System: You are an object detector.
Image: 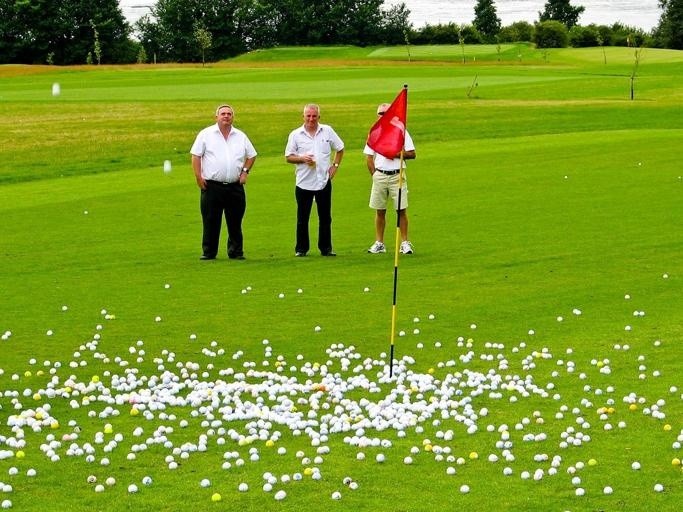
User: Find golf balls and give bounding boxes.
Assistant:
[1,271,682,509]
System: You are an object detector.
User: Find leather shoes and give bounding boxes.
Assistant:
[320,251,336,256]
[294,252,305,256]
[199,256,215,260]
[229,256,245,260]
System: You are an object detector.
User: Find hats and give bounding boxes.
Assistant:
[376,103,390,114]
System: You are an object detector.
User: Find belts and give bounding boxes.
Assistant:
[206,180,239,186]
[375,168,399,175]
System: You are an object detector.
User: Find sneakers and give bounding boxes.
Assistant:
[399,241,413,254]
[367,243,385,254]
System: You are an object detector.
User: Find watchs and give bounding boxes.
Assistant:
[333,162,339,168]
[242,167,249,174]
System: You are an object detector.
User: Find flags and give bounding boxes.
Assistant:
[367,87,405,160]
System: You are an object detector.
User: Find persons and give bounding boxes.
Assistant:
[363,102,416,253]
[284,103,345,257]
[190,105,257,260]
